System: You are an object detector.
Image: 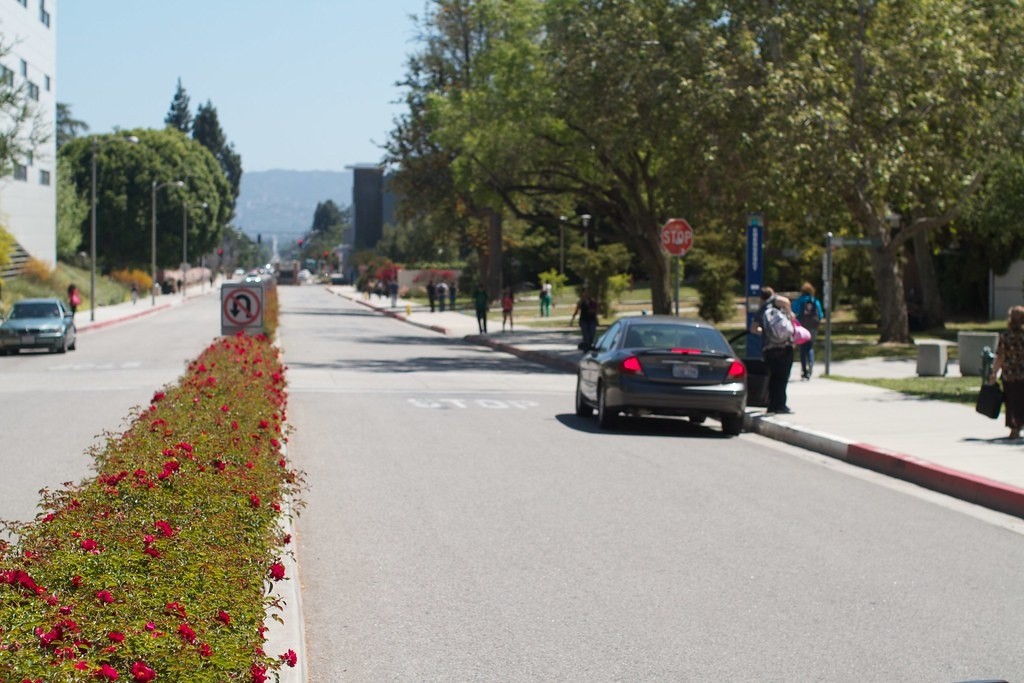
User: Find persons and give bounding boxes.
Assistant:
[751,287,795,414]
[68,284,78,316]
[502,286,514,332]
[989,306,1024,439]
[539,279,552,318]
[473,283,490,334]
[427,280,456,307]
[574,288,598,353]
[791,283,823,380]
[131,283,138,304]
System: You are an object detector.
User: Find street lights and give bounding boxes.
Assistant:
[559,216,568,274]
[152,180,184,306]
[90,136,139,322]
[582,214,591,249]
[182,203,208,294]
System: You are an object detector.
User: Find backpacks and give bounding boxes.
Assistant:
[758,297,794,343]
[796,296,820,328]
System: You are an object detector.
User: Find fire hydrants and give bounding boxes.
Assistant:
[980,346,997,383]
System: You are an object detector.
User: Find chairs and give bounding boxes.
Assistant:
[625,332,646,347]
[680,335,700,348]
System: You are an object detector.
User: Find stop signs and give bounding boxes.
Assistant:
[660,218,693,256]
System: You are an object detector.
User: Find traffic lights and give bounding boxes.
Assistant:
[218,249,223,256]
[299,238,302,248]
[324,252,329,257]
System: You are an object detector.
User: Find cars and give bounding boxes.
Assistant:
[0,298,76,358]
[574,317,769,436]
[234,269,276,282]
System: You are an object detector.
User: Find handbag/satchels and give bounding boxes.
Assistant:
[976,372,1004,419]
[790,317,811,345]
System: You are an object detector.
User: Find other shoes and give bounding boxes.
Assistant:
[767,406,795,414]
